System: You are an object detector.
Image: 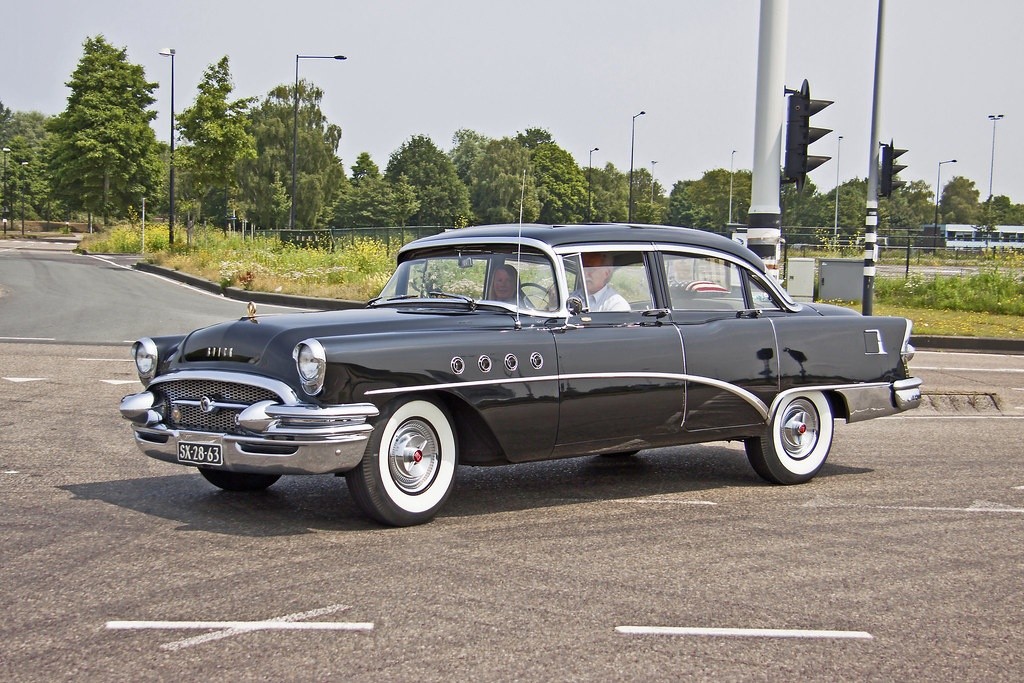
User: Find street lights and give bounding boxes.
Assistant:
[651,160,657,205]
[289,55,349,230]
[933,159,958,257]
[628,111,645,223]
[728,149,737,224]
[835,136,843,244]
[588,148,599,222]
[3,148,11,234]
[986,114,1005,256]
[22,162,28,235]
[158,48,175,245]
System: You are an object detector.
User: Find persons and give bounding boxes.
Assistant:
[545,252,631,313]
[489,264,527,307]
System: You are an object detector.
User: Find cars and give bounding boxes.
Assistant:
[117,224,923,528]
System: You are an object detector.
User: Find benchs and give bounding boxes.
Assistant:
[650,299,745,308]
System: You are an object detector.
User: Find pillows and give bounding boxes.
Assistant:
[669,279,730,295]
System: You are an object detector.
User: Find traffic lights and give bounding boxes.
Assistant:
[880,138,908,199]
[787,79,835,194]
